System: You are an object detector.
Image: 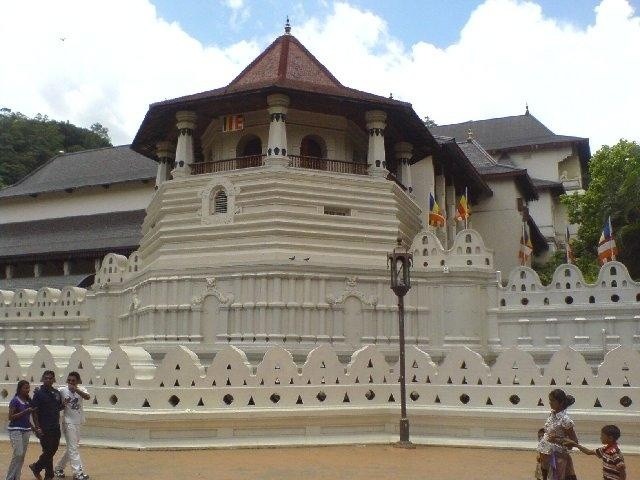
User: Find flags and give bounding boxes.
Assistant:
[429,192,445,229]
[565,229,576,259]
[520,227,533,265]
[597,219,616,261]
[457,193,472,221]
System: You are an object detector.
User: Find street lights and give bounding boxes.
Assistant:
[386,236,415,450]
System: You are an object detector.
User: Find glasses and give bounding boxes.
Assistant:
[67,378,75,382]
[44,377,53,380]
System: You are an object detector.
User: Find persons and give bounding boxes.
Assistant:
[6,380,38,480]
[30,371,90,480]
[563,424,627,480]
[536,389,579,480]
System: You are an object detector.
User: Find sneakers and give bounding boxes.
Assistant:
[72,474,88,480]
[29,463,65,480]
